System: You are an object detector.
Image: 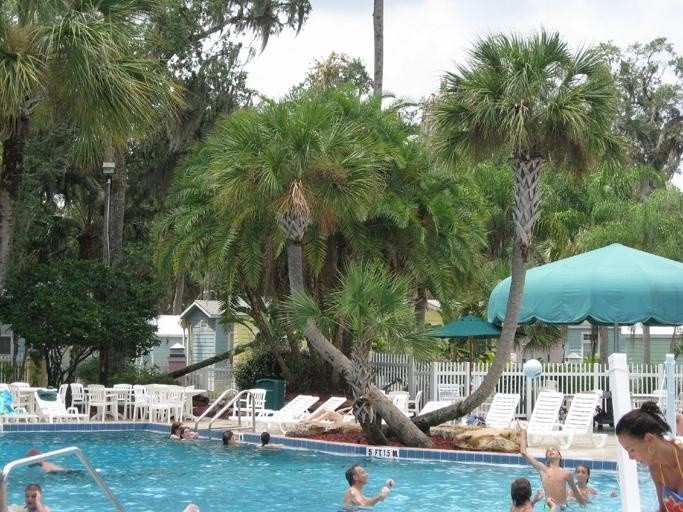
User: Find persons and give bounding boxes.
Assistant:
[169,421,183,440]
[507,477,545,512]
[179,427,199,441]
[614,402,683,511]
[569,463,617,502]
[25,449,85,476]
[222,431,238,444]
[515,416,588,510]
[7,483,51,511]
[259,432,283,448]
[309,406,360,424]
[342,464,393,507]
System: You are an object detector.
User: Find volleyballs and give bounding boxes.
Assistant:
[523,359,542,378]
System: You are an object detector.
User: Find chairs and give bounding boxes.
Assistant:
[0,378,207,430]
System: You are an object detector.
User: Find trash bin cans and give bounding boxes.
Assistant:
[256,378,284,410]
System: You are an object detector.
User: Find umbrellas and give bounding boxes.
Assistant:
[486,238,681,352]
[416,310,526,398]
